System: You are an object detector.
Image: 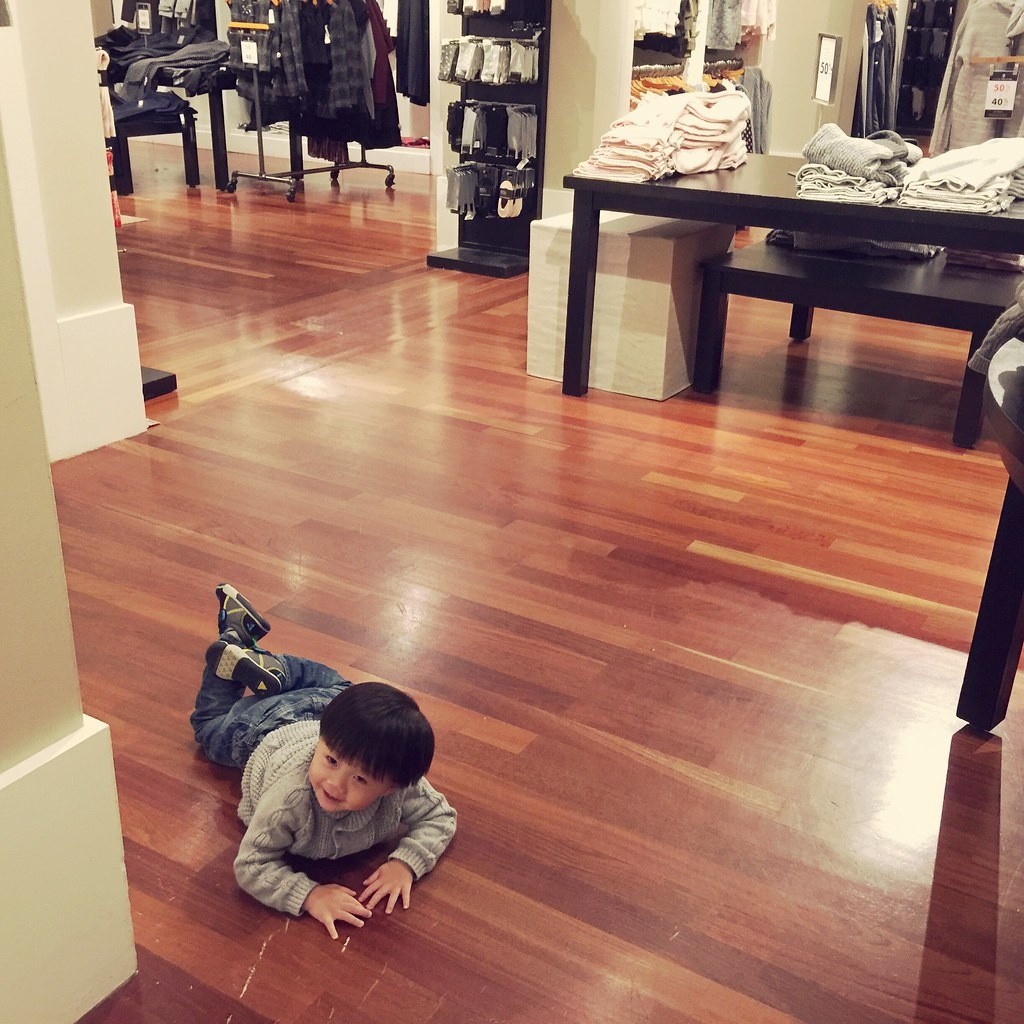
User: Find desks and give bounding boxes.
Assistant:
[561,151,1024,398]
[108,59,305,192]
[954,328,1024,734]
[105,92,202,195]
[693,234,1024,450]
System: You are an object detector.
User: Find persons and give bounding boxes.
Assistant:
[189,581,456,940]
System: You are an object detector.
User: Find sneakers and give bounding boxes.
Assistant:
[215,583,271,650]
[205,638,288,698]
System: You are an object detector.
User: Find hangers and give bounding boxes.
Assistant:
[630,57,760,113]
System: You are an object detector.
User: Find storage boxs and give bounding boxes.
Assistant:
[524,210,737,402]
